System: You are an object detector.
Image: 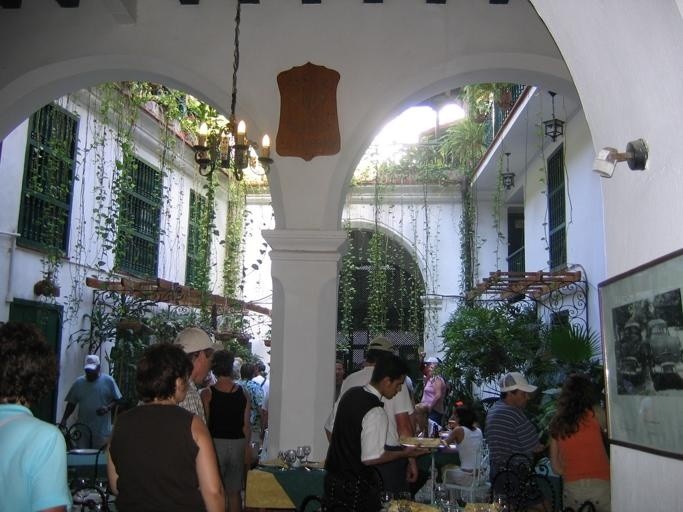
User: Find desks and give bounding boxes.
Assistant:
[397,437,447,482]
[256,464,327,511]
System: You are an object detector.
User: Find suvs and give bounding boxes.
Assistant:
[646,318,680,364]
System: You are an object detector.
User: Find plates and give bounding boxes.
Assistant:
[404,443,445,448]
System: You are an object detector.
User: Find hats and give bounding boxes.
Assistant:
[82,354,102,371]
[364,334,396,356]
[496,369,539,397]
[173,327,225,355]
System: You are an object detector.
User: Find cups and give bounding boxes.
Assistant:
[494,495,507,512]
[434,483,449,511]
[303,445,311,465]
[287,450,297,468]
[379,491,394,511]
[397,491,411,512]
[296,446,305,469]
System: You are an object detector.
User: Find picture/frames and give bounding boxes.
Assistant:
[597,249,683,462]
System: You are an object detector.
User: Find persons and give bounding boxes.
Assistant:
[548,376,610,512]
[234,364,265,491]
[394,348,416,408]
[415,403,443,438]
[171,328,213,426]
[201,350,255,512]
[106,342,228,512]
[341,337,418,500]
[0,320,73,512]
[419,352,446,427]
[232,359,241,384]
[484,372,551,512]
[59,355,123,450]
[254,363,269,437]
[234,357,244,369]
[335,358,346,403]
[324,355,432,512]
[448,401,464,430]
[442,406,484,512]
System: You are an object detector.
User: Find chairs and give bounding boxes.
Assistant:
[56,422,69,450]
[437,445,490,510]
[498,450,565,511]
[69,420,91,450]
[94,440,110,483]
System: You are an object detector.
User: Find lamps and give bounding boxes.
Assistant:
[499,148,516,192]
[543,90,568,141]
[592,137,651,178]
[191,1,273,182]
